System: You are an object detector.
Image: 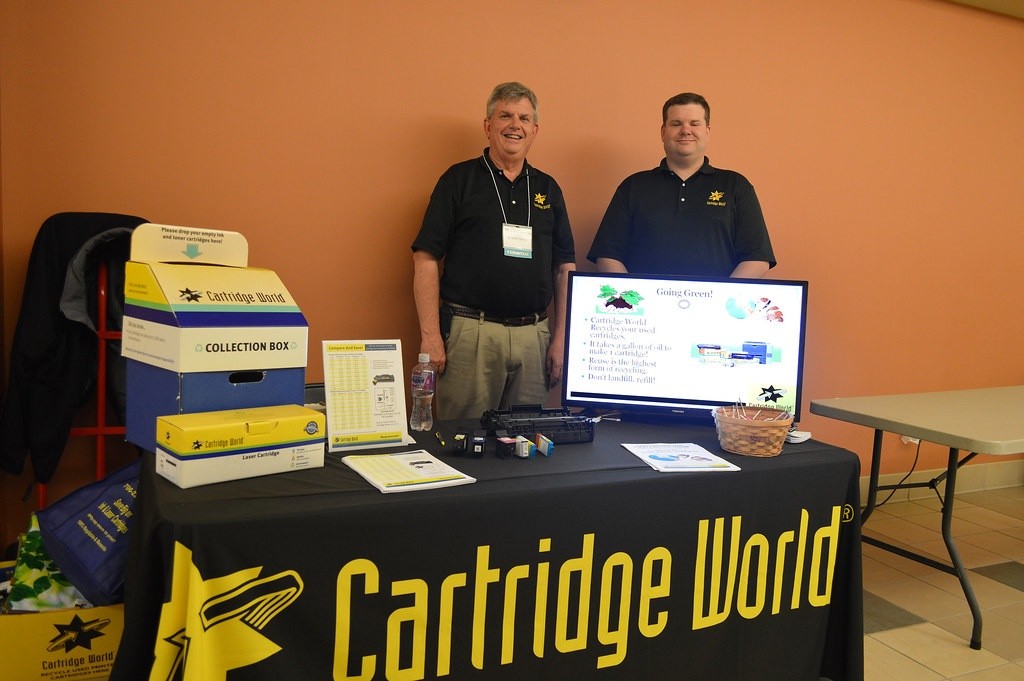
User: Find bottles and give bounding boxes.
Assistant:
[409,353,436,431]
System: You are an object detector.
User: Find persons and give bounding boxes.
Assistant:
[411,82,577,421]
[585,93,777,279]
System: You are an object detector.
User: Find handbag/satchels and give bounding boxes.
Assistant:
[35,461,139,606]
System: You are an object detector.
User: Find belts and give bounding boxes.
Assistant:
[448,305,548,327]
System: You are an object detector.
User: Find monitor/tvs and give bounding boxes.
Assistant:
[561,270,809,426]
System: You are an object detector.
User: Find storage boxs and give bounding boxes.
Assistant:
[123,225,327,490]
[0,603,125,681]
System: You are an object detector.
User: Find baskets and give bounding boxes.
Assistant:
[712,406,795,457]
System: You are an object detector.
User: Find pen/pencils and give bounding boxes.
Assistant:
[435,432,446,446]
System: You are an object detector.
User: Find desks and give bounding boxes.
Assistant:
[106,414,865,681]
[809,385,1024,651]
